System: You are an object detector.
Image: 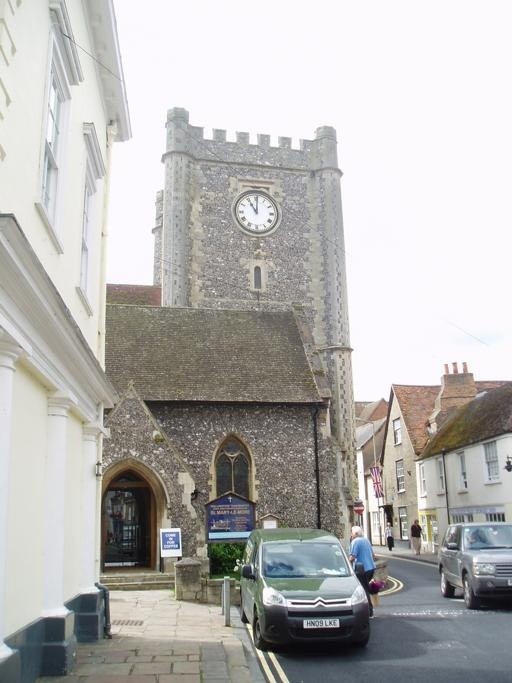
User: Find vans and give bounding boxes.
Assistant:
[235,524,372,650]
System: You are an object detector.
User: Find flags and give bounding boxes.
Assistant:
[369,466,385,496]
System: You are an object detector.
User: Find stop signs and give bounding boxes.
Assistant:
[353,502,365,514]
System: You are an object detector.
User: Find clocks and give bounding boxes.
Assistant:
[230,189,282,238]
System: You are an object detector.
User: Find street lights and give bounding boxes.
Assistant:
[355,416,384,547]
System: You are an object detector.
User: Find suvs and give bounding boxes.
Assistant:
[435,521,512,607]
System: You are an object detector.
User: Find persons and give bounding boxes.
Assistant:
[384,521,394,551]
[409,519,422,555]
[464,531,486,547]
[347,525,376,622]
